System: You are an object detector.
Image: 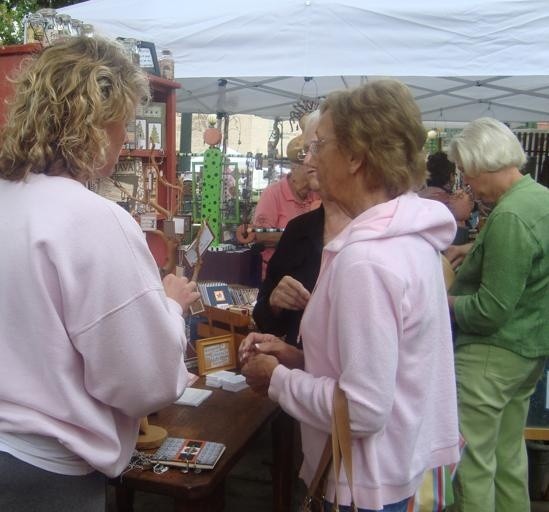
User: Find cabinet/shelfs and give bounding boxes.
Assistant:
[194,303,252,351]
[0,43,184,279]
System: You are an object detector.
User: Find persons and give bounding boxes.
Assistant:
[0,33,200,512]
[233,78,549,512]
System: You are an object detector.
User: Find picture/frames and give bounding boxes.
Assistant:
[195,332,237,375]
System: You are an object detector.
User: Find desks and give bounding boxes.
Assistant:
[105,372,291,512]
[179,242,264,289]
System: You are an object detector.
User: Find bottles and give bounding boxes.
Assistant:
[160,50,175,81]
[122,37,141,65]
[24,7,95,46]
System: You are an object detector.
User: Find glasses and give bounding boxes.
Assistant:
[297,143,320,162]
